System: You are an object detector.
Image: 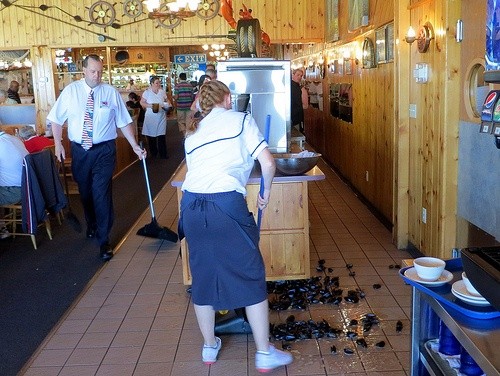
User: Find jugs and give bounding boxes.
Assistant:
[151,103,159,113]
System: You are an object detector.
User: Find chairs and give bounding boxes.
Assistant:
[0,125,73,251]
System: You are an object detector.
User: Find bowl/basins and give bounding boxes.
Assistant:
[413,256,446,281]
[462,271,482,296]
[438,318,484,376]
[162,106,175,111]
[271,152,322,175]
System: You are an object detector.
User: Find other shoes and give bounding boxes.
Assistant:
[86,226,94,237]
[100,241,113,260]
[0,221,10,239]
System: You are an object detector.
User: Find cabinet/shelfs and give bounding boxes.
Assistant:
[400,258,500,376]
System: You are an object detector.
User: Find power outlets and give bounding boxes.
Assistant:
[422,207,427,224]
[365,170,368,182]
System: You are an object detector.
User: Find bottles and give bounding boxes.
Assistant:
[14,128,19,138]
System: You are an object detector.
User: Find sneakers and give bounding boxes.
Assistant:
[201,335,221,364]
[256,345,292,372]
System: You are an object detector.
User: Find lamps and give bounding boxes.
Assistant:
[142,0,201,19]
[404,26,430,53]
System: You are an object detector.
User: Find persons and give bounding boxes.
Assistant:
[47,54,146,260]
[126,93,145,128]
[179,80,293,374]
[0,121,30,238]
[126,79,140,92]
[291,70,308,129]
[139,77,172,160]
[190,64,216,117]
[0,90,18,104]
[18,125,53,153]
[174,73,193,135]
[7,81,21,104]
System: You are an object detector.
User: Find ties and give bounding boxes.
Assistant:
[81,89,94,151]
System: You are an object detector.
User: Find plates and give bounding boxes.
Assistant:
[450,280,490,307]
[404,267,454,287]
